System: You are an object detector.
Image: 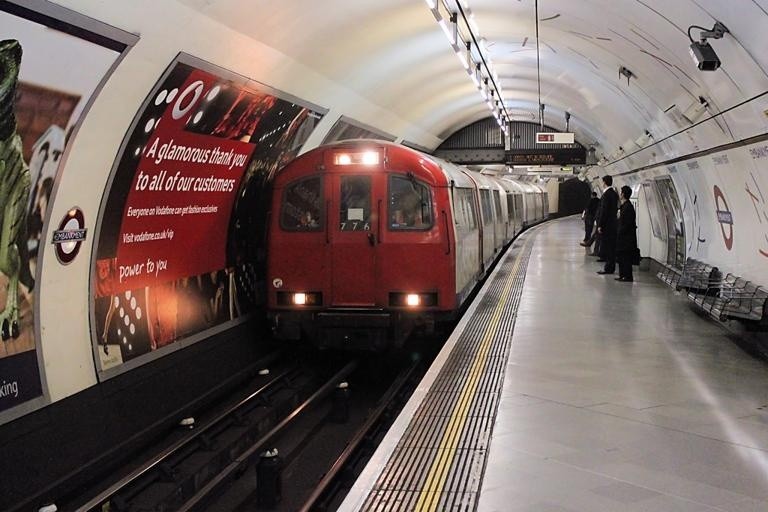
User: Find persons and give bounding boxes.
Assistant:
[580,191,600,241]
[580,228,600,256]
[596,175,619,275]
[390,189,428,230]
[614,184,637,282]
[200,269,227,325]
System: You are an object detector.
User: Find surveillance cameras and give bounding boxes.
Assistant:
[688,40,722,72]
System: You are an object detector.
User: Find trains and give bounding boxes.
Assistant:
[266,138,549,351]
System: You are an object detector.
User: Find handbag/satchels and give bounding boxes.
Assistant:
[615,247,640,265]
[580,237,594,246]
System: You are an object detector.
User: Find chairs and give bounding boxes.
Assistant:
[656,256,767,333]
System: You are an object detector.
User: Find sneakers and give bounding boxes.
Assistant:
[589,251,633,281]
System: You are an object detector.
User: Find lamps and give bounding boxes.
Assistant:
[580,133,652,175]
[682,96,709,124]
[425,0,510,137]
